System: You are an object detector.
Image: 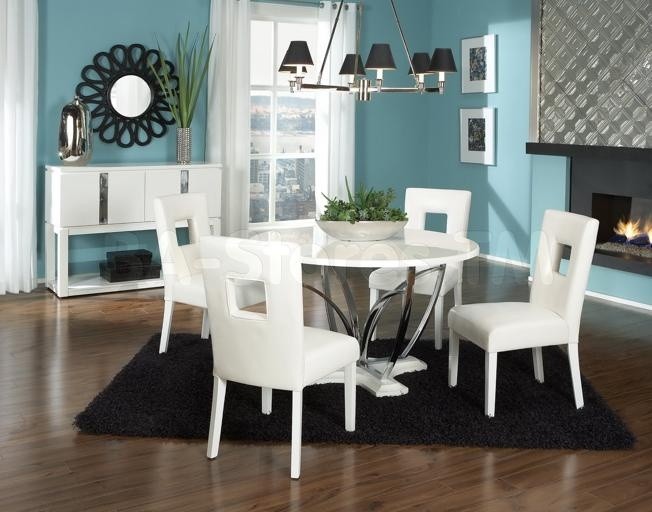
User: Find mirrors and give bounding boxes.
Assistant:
[73,43,183,148]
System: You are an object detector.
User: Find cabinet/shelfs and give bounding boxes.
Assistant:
[43,158,225,301]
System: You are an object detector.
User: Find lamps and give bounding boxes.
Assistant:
[274,0,460,105]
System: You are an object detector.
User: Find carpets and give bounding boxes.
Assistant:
[66,324,639,460]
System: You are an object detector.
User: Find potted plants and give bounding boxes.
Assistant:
[145,16,225,167]
[308,175,411,244]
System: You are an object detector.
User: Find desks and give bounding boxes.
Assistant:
[244,222,482,403]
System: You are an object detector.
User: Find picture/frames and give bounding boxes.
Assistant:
[456,107,500,169]
[454,32,497,96]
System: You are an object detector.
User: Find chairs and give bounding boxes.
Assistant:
[191,233,364,480]
[359,183,476,350]
[439,205,603,420]
[144,188,226,357]
